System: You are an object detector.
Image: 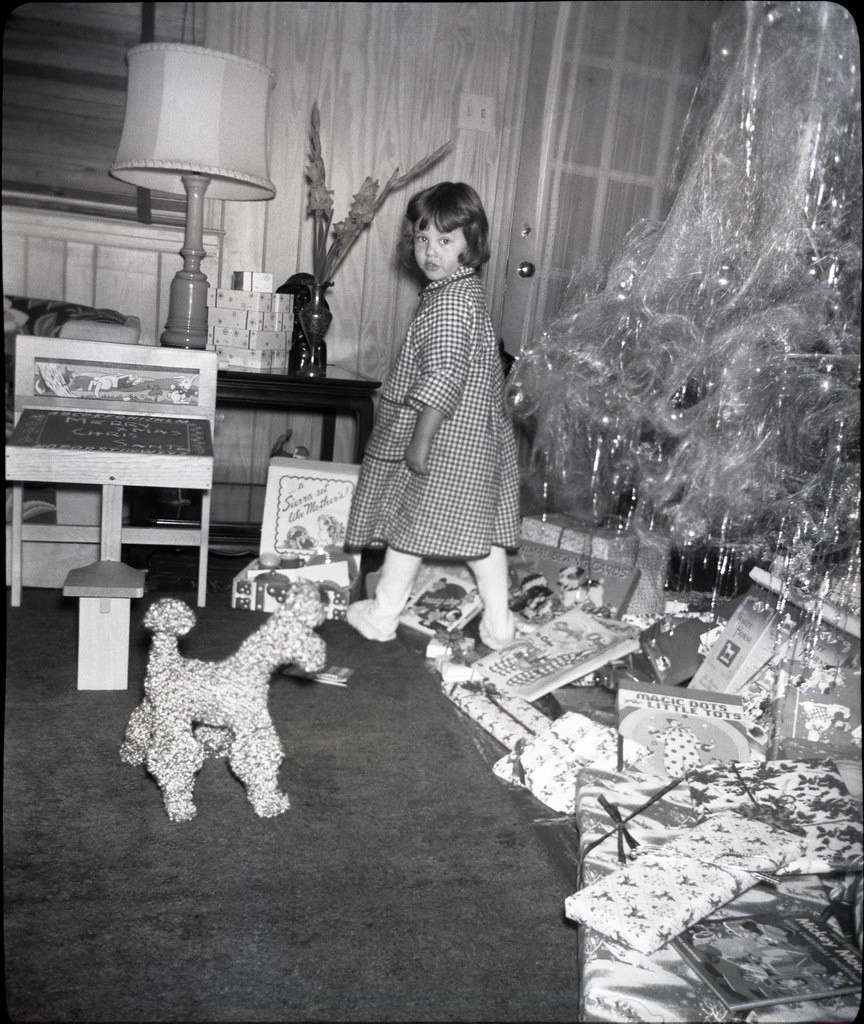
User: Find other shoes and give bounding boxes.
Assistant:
[344,598,397,642]
[478,608,516,650]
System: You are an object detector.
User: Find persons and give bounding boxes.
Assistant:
[343,180,525,652]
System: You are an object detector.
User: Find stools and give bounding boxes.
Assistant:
[5,409,213,608]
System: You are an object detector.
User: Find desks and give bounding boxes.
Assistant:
[124,360,386,587]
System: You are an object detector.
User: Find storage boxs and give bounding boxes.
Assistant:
[421,601,864,1024]
[229,458,361,622]
[206,272,294,373]
[524,515,674,618]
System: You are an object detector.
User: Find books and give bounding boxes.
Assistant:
[687,591,858,720]
[470,604,642,704]
[613,676,752,773]
[398,573,485,641]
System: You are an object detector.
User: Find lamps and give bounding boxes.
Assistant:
[109,43,278,349]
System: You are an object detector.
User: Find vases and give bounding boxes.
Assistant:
[298,282,335,377]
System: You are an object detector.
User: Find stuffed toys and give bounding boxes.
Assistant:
[554,564,606,612]
[118,583,328,822]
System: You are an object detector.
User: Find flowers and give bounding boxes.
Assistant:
[300,102,454,283]
